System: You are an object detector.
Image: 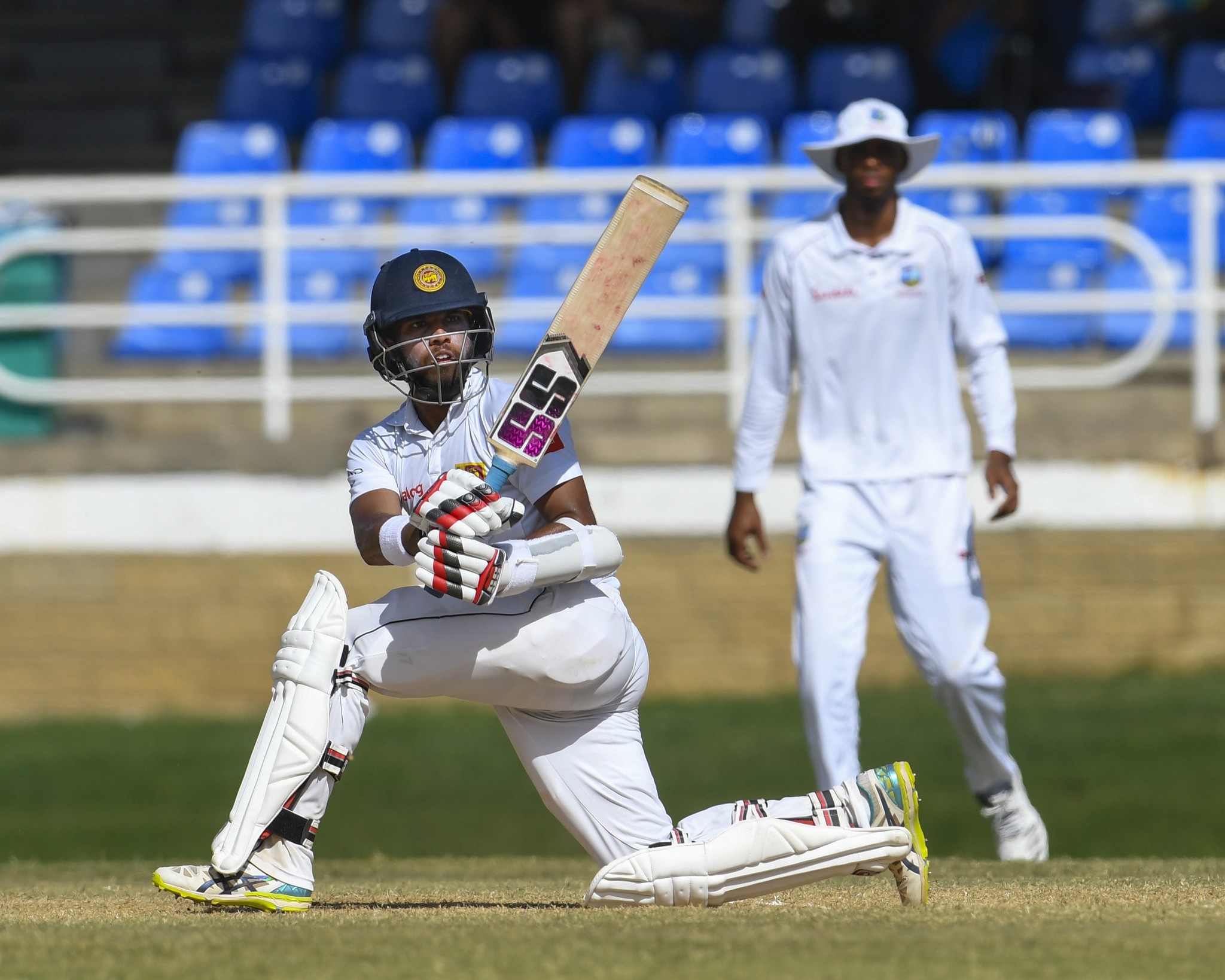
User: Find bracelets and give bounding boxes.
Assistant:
[379,516,416,567]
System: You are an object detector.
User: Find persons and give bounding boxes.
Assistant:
[727,99,1049,861]
[152,248,929,912]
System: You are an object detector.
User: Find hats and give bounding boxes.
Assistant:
[804,98,941,185]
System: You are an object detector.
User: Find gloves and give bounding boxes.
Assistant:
[414,529,506,608]
[408,469,513,538]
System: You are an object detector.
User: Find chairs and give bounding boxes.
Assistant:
[100,2,1224,365]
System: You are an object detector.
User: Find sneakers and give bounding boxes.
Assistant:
[858,761,932,913]
[977,752,1048,865]
[150,858,312,916]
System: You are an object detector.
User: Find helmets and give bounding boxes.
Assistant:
[362,248,492,380]
